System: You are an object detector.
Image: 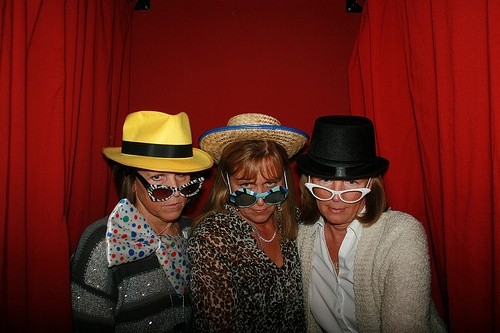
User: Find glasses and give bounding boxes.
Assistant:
[305,174,372,203]
[227,171,289,206]
[129,170,205,201]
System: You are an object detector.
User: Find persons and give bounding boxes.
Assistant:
[68,110,215,333]
[185,112,311,333]
[294,114,451,333]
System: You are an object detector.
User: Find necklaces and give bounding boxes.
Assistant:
[250,225,277,243]
[151,222,172,236]
[323,224,340,271]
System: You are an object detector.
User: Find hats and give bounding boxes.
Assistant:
[302,116,389,179]
[103,110,213,173]
[198,112,309,166]
